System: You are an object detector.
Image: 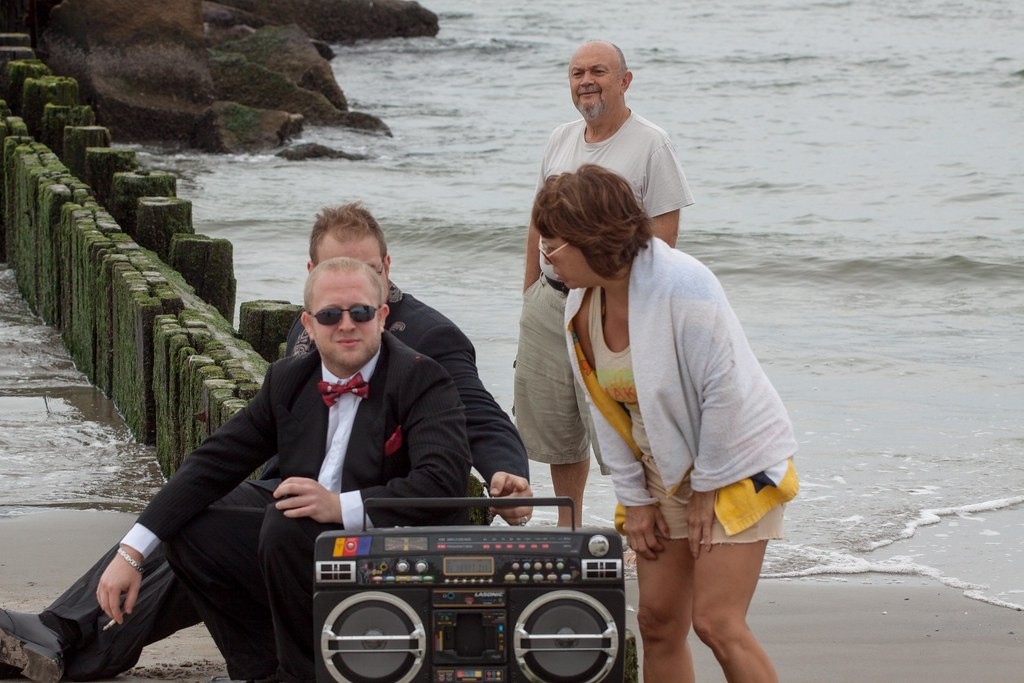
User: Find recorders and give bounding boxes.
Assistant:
[312,497,630,683]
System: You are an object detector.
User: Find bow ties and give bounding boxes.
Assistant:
[316,371,369,408]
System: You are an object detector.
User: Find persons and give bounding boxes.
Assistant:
[534,163,799,683]
[509,38,697,528]
[97,258,473,683]
[1,203,533,683]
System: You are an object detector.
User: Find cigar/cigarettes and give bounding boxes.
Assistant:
[101,610,126,630]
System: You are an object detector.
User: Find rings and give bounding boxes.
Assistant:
[520,516,527,526]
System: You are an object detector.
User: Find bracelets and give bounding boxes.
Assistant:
[116,547,146,576]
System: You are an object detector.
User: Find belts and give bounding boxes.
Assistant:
[545,274,569,296]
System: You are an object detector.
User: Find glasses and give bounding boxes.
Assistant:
[538,242,568,264]
[313,303,378,325]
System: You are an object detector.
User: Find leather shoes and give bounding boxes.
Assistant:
[0,603,74,683]
